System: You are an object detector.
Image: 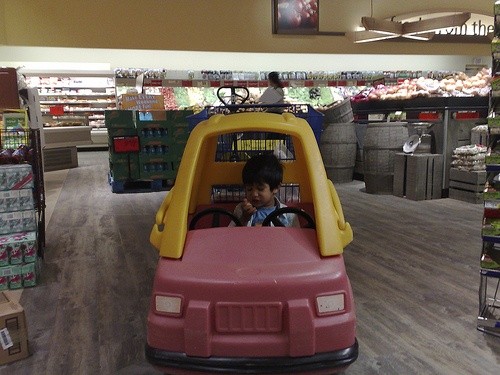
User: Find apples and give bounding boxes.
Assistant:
[368,67,491,101]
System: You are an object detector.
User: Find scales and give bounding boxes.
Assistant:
[402,121,432,153]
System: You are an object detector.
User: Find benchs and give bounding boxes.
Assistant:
[193,202,317,229]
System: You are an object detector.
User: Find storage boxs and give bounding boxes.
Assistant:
[0,289,30,365]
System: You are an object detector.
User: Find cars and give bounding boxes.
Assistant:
[139,110,360,367]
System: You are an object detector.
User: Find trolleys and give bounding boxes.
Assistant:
[217,84,250,112]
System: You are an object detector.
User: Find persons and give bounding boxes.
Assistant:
[261,72,284,114]
[227,154,300,230]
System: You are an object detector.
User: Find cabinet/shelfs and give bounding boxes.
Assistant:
[0,0,500,337]
